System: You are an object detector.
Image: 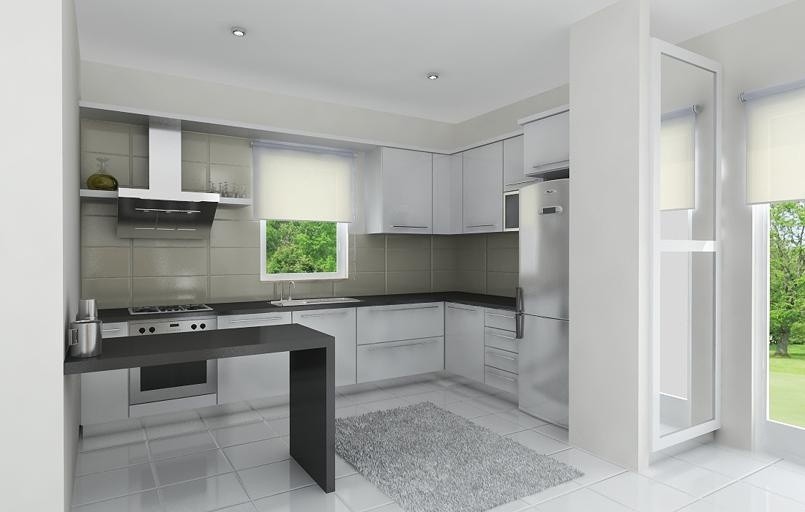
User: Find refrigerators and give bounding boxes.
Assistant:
[514,177,569,432]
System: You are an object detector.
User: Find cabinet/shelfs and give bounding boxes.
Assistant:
[517,104,571,179]
[485,327,520,356]
[357,335,444,384]
[357,145,434,235]
[484,346,519,376]
[484,365,519,395]
[445,302,485,383]
[80,321,128,426]
[217,312,292,405]
[432,152,464,234]
[485,306,517,333]
[502,134,545,196]
[292,307,356,387]
[356,301,445,346]
[462,140,504,234]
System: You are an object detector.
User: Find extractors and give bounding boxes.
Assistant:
[116,187,220,241]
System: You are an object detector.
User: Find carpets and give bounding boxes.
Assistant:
[334,400,585,512]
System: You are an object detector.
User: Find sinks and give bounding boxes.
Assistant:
[268,298,361,306]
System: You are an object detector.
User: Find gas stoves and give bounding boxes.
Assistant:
[132,306,157,313]
[182,304,205,310]
[158,305,180,312]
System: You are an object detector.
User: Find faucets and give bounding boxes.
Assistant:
[287,280,295,302]
